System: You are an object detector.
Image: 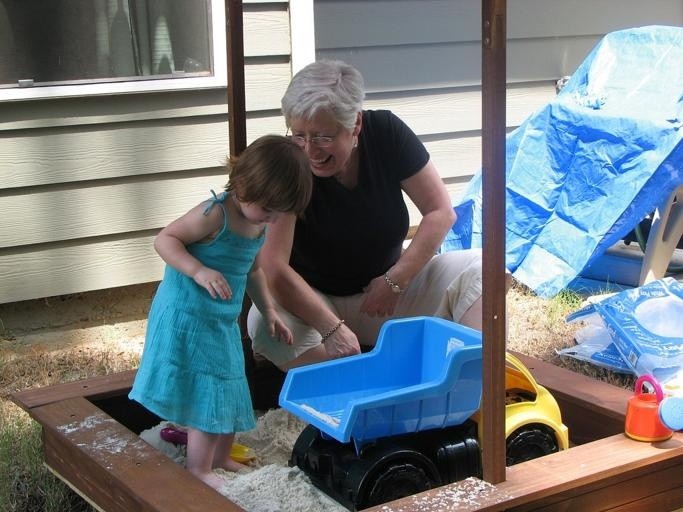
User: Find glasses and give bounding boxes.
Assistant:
[286,125,342,148]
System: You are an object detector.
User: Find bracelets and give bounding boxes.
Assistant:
[321,315,347,341]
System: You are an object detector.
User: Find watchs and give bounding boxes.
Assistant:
[384,274,404,297]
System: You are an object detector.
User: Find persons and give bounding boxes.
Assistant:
[128,134,318,476]
[242,57,512,392]
